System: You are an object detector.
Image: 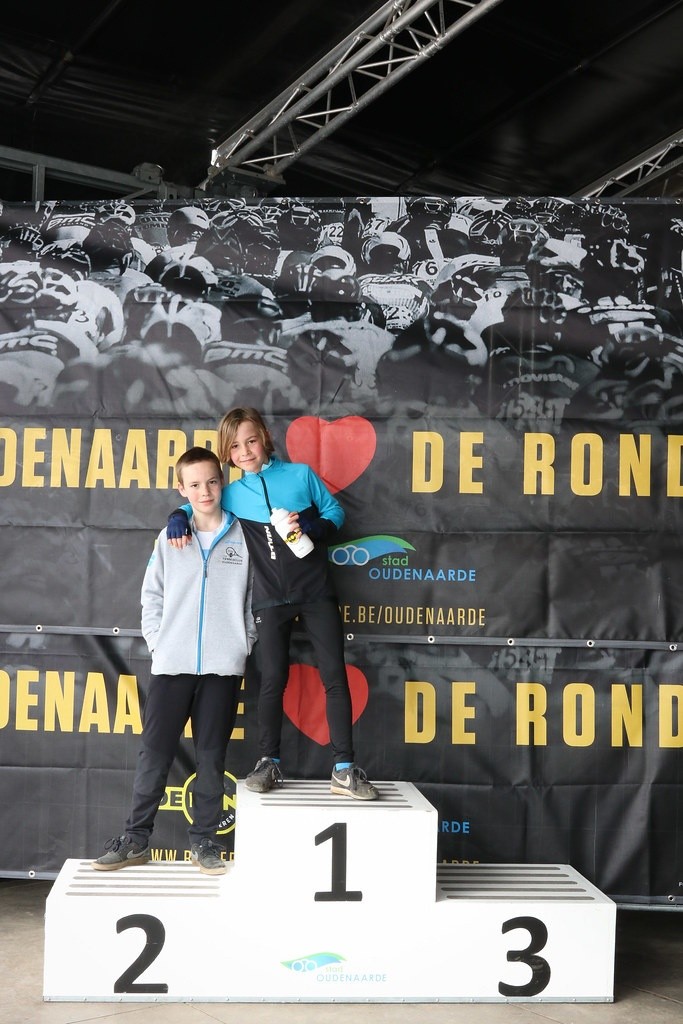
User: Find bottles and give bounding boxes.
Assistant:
[268,506,315,558]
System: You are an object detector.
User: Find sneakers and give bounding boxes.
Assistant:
[330,766,379,800]
[245,760,278,792]
[93,836,149,871]
[190,842,226,875]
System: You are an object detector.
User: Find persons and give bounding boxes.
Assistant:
[1,194,682,439]
[164,405,379,802]
[94,447,258,872]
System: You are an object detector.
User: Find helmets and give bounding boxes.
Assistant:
[0,201,682,428]
